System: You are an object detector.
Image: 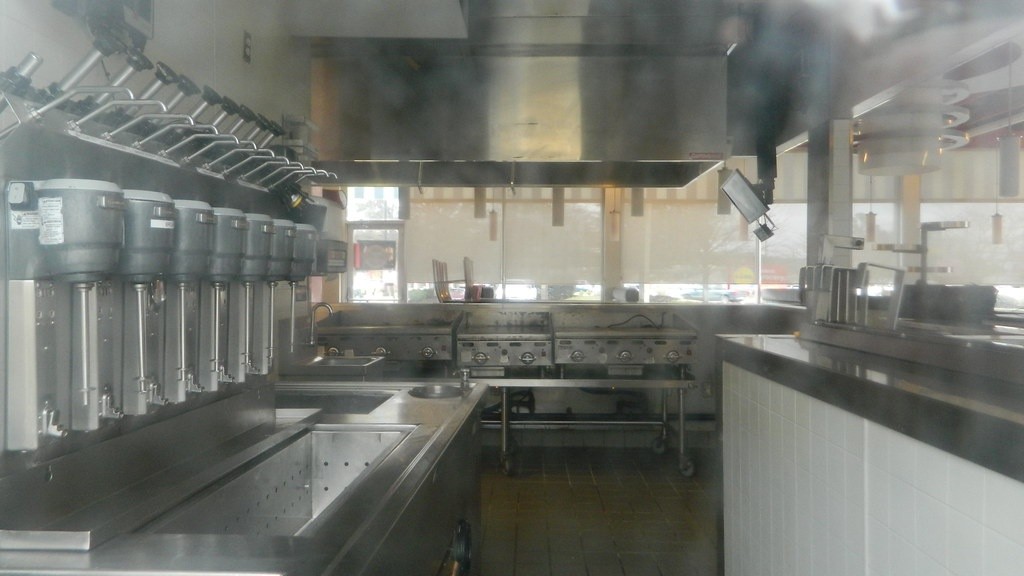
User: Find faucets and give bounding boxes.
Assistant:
[310,301,334,348]
[452,367,471,391]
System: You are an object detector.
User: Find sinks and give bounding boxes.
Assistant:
[407,382,463,401]
[132,423,420,539]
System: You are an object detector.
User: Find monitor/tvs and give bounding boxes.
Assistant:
[723,168,767,226]
[356,239,396,271]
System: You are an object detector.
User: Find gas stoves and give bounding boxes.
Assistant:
[549,301,698,368]
[453,309,552,368]
[315,304,462,362]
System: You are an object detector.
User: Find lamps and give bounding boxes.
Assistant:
[861,110,943,174]
